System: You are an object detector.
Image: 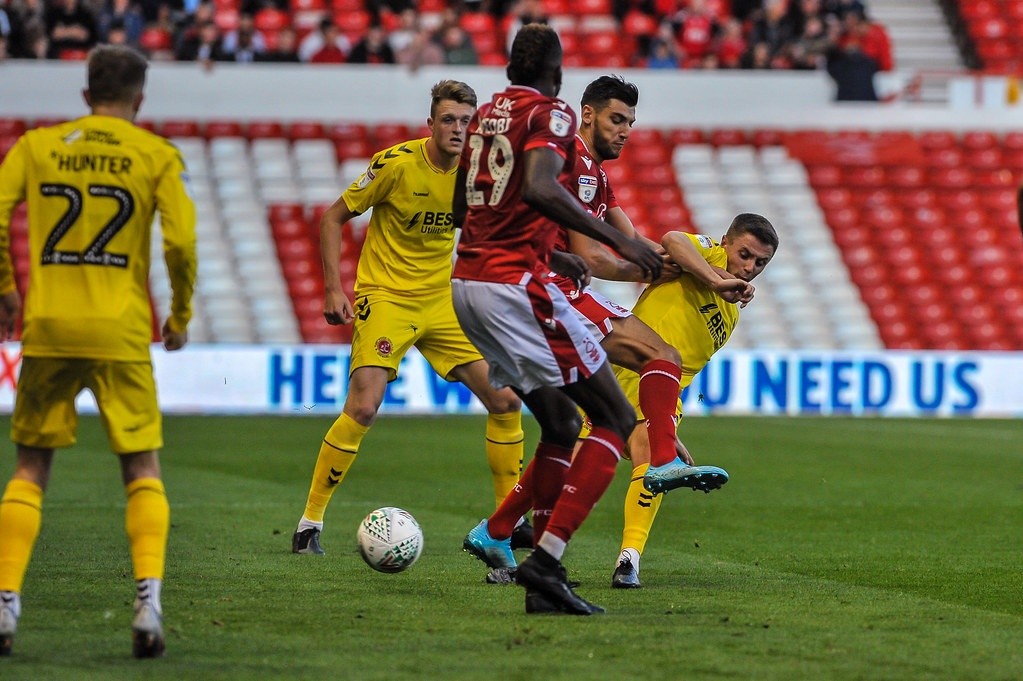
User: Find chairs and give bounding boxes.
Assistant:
[60,0,1023,74]
[0,114,1023,352]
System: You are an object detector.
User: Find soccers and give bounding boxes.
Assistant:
[357,507,424,574]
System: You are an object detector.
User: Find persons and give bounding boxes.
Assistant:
[465,73,730,583]
[0,47,196,660]
[289,79,535,557]
[452,23,662,618]
[0,0,899,105]
[573,212,780,589]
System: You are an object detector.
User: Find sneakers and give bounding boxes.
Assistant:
[463,519,517,569]
[131,603,166,659]
[612,558,641,588]
[643,456,729,497]
[293,527,326,556]
[487,569,516,584]
[516,552,593,615]
[526,589,605,615]
[0,595,20,654]
[511,519,539,549]
